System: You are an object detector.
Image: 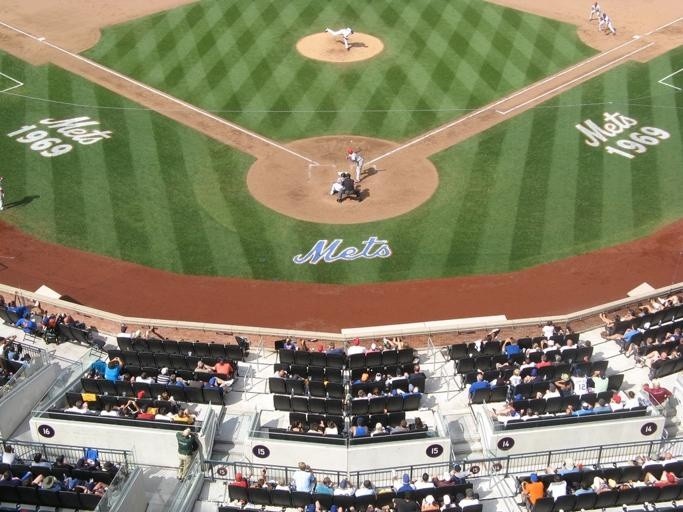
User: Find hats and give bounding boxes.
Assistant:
[644,322,650,329]
[161,367,169,375]
[531,474,538,482]
[340,480,346,488]
[653,381,659,386]
[615,396,621,402]
[209,378,215,385]
[403,465,461,483]
[562,373,570,381]
[56,455,116,472]
[138,391,145,398]
[183,428,191,436]
[306,504,337,512]
[236,473,242,480]
[425,489,474,505]
[44,476,54,489]
[666,473,675,483]
[316,339,377,352]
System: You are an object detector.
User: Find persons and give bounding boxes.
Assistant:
[469,321,640,425]
[272,339,428,438]
[330,172,346,195]
[336,173,362,202]
[598,13,616,35]
[0,294,236,498]
[517,454,680,509]
[599,294,682,367]
[325,27,353,48]
[589,2,600,20]
[347,149,364,181]
[232,461,479,511]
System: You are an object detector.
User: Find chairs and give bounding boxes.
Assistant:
[0,286,97,387]
[1,461,116,509]
[47,336,247,435]
[446,331,648,432]
[267,346,429,449]
[514,458,682,510]
[608,303,682,381]
[218,479,486,510]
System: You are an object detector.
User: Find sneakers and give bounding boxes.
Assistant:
[518,503,526,506]
[225,379,234,393]
